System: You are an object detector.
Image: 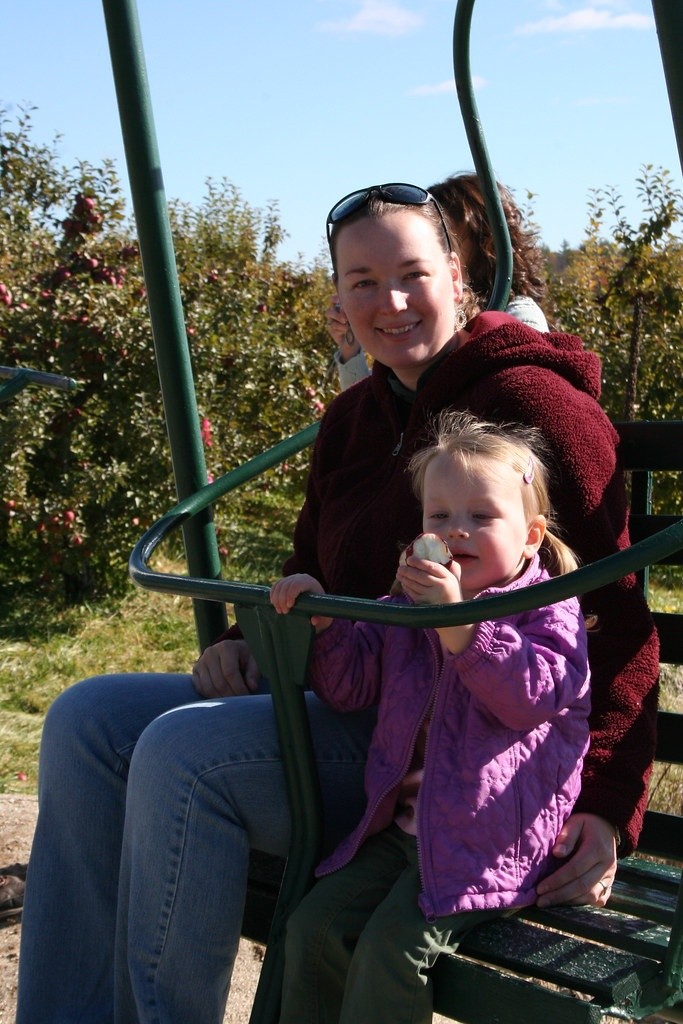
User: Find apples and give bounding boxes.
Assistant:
[397,532,453,571]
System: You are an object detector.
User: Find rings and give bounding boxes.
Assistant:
[600,880,608,895]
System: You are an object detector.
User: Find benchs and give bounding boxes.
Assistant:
[237,426,683,1024]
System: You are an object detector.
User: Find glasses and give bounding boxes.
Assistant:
[325,183,452,256]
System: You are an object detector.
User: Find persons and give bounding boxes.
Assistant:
[326,172,550,393]
[270,411,590,1024]
[13,183,661,1024]
